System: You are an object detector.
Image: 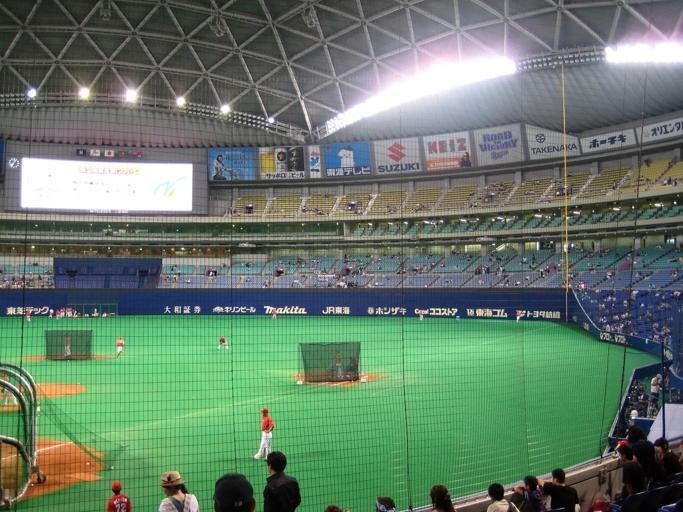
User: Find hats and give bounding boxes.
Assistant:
[258,407,268,414]
[110,480,123,492]
[158,470,186,490]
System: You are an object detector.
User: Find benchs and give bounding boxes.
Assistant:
[351,205,683,237]
[1,219,344,240]
[230,156,683,219]
[0,263,51,289]
[160,246,683,289]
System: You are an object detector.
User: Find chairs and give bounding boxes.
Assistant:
[575,291,683,348]
[604,471,683,511]
[0,153,683,290]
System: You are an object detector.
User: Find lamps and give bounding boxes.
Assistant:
[204,9,226,40]
[98,2,114,22]
[300,4,317,31]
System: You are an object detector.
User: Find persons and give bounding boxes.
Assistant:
[211,155,232,180]
